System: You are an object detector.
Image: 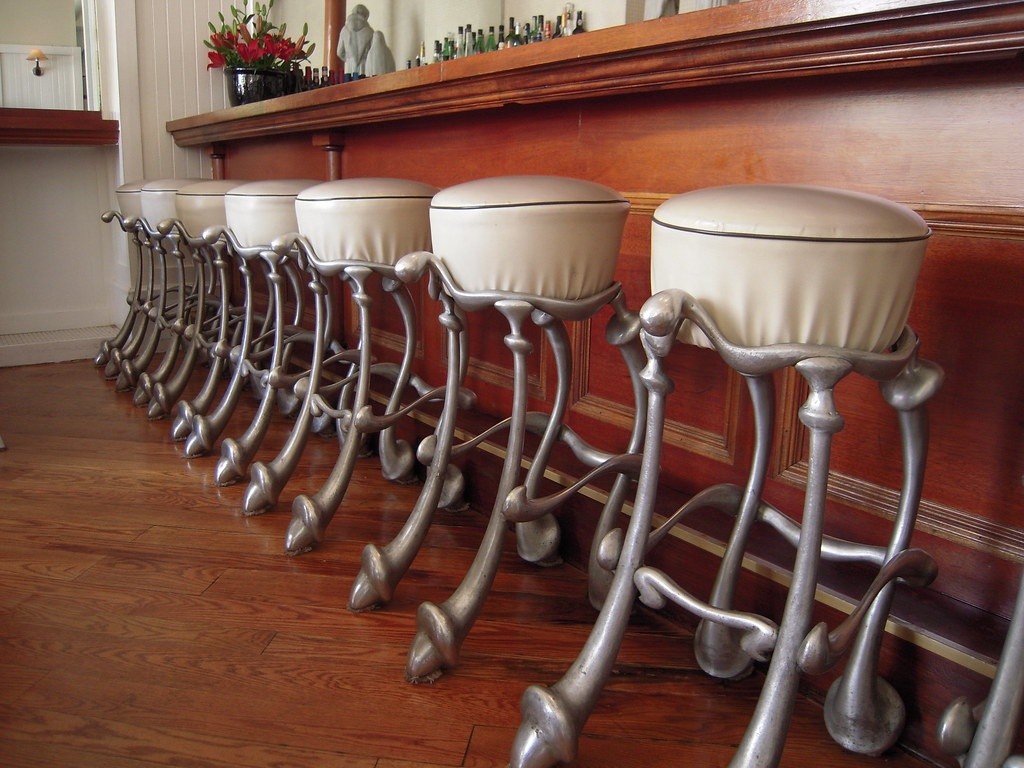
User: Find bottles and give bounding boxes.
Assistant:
[292,4,586,91]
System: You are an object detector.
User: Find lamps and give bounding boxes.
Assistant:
[24,48,48,76]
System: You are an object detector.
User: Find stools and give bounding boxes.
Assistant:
[96,175,946,768]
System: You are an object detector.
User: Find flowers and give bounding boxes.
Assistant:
[203,0,316,76]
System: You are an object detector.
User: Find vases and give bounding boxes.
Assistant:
[224,66,294,106]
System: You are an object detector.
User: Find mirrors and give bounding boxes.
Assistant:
[0,0,101,112]
